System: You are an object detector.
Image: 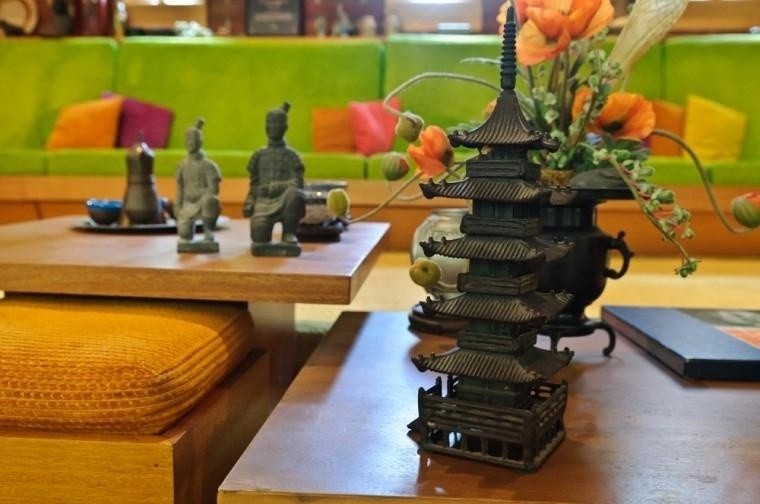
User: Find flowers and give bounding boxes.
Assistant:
[326,0,760,279]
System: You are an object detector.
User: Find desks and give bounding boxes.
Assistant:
[0,213,392,305]
[216,310,760,504]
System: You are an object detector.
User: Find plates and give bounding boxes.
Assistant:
[69,215,231,232]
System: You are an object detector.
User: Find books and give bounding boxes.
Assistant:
[601,306,760,381]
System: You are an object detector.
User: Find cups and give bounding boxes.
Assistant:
[86,198,123,226]
[303,180,347,192]
[299,190,335,223]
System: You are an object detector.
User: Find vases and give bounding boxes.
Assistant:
[534,187,641,359]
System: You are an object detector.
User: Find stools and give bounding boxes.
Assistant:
[0,294,273,504]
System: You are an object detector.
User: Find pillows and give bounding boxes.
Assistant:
[44,95,125,150]
[643,97,687,157]
[347,96,401,158]
[682,95,748,162]
[311,107,356,153]
[100,92,176,150]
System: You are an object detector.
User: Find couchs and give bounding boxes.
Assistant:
[0,33,759,258]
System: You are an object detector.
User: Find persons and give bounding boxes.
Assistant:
[175,119,221,240]
[242,100,307,244]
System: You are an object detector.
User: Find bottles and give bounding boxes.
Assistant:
[411,207,473,285]
[123,129,162,224]
